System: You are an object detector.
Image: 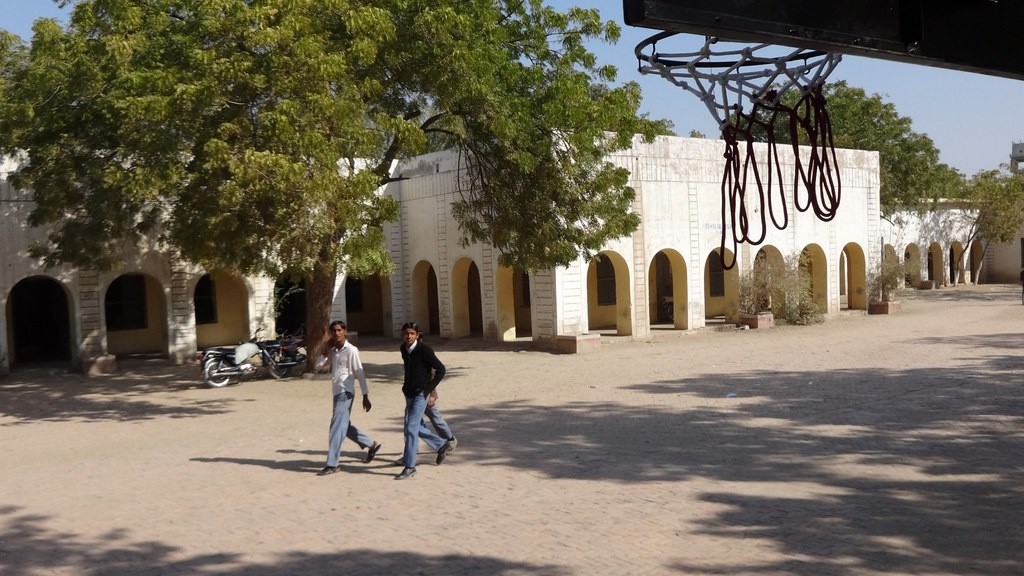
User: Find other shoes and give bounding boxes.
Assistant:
[366,441,382,463]
[448,436,457,454]
[319,466,341,476]
[394,457,405,465]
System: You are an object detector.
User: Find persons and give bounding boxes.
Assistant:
[394,322,458,480]
[316,320,381,475]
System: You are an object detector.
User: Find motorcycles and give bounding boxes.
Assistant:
[254,327,308,389]
[197,325,273,388]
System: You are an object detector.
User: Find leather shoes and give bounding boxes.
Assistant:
[397,467,417,479]
[436,441,451,464]
